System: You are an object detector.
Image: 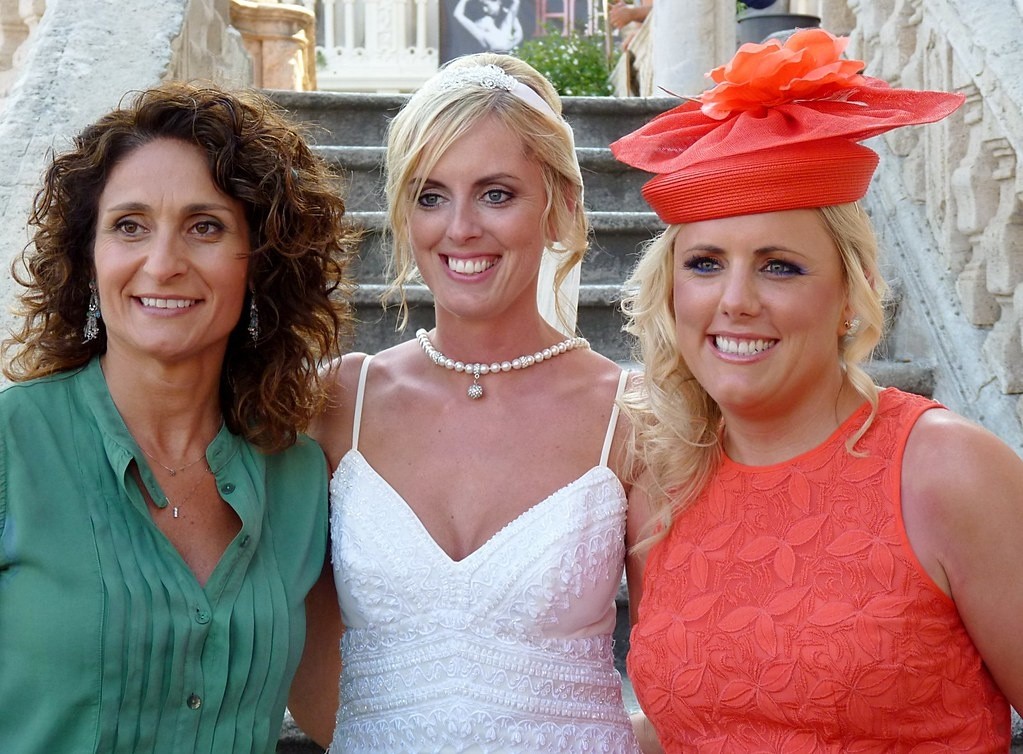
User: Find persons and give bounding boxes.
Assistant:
[623,140,1023,754]
[607,0,653,52]
[303,54,645,754]
[0,83,346,754]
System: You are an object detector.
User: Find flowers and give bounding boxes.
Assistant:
[699,27,865,120]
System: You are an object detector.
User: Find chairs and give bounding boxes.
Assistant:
[229,0,318,91]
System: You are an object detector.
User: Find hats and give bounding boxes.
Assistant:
[609,27,966,225]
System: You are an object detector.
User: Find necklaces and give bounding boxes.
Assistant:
[414,328,589,400]
[137,444,211,517]
